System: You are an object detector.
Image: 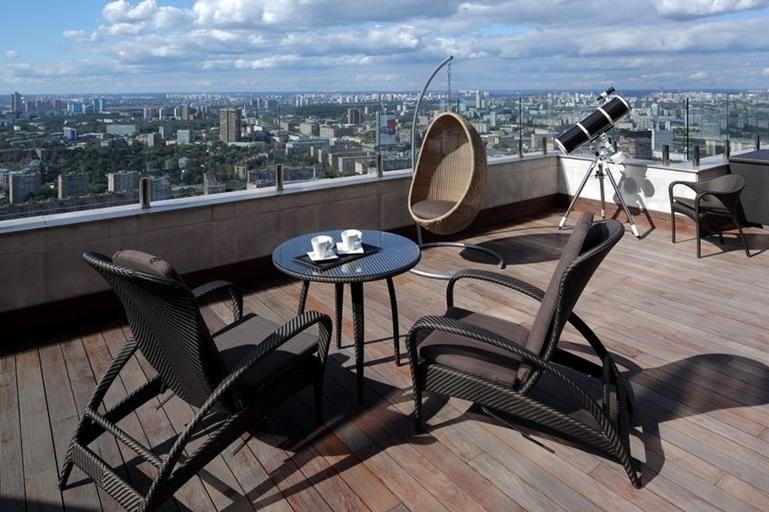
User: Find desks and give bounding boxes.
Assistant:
[272,228,420,407]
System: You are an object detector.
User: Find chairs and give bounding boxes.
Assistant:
[405,211,641,489]
[669,174,751,258]
[60,250,333,512]
[408,111,488,236]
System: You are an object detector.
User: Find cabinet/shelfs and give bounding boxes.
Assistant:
[727,150,769,225]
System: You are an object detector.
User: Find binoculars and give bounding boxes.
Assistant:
[553,87,632,155]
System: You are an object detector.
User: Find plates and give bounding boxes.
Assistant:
[336,242,364,255]
[306,251,339,262]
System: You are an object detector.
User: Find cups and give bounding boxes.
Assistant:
[310,235,333,257]
[340,230,363,250]
